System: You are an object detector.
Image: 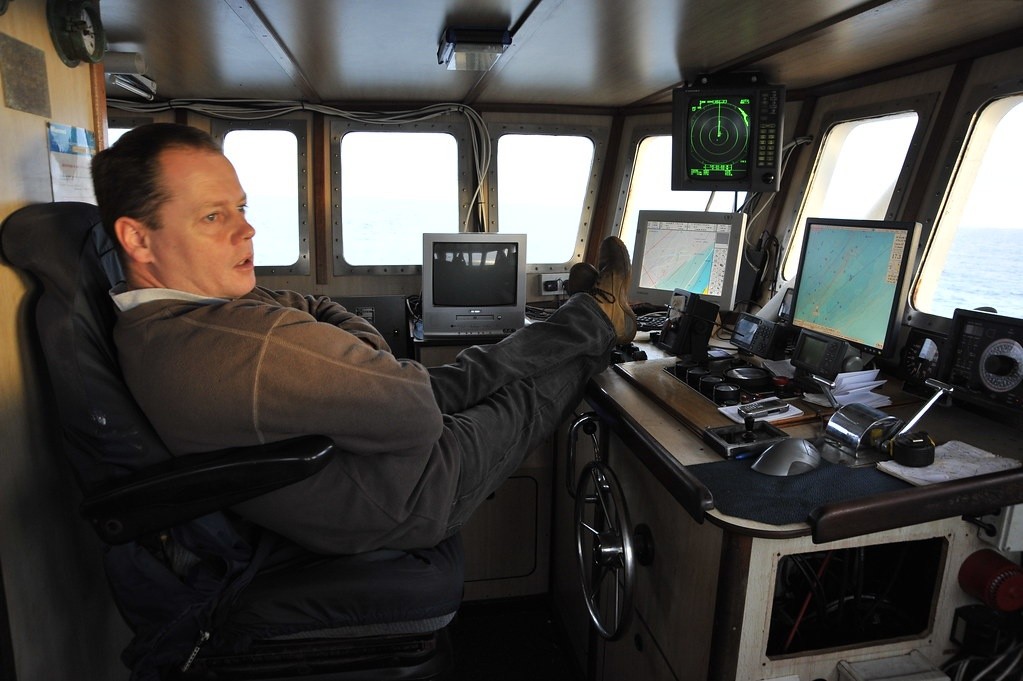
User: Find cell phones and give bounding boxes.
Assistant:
[738,399,789,419]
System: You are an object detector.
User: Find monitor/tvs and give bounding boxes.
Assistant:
[628,210,747,311]
[789,217,922,359]
[687,95,754,181]
[423,233,527,337]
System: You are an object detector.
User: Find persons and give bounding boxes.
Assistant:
[90,123,637,556]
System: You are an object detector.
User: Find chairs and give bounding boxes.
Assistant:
[0,200,467,681]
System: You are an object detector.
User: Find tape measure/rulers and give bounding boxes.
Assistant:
[889,431,936,468]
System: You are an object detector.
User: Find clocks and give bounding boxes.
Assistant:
[62,1,109,63]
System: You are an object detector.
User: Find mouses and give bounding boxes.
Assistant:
[750,437,822,476]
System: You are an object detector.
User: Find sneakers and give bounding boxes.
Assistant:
[567,263,599,298]
[583,236,637,345]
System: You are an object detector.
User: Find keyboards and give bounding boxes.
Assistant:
[636,317,667,330]
